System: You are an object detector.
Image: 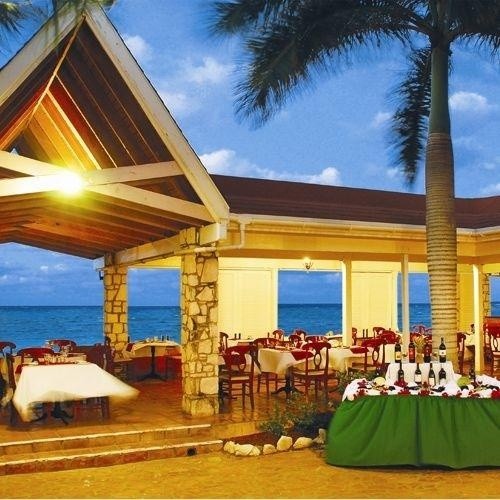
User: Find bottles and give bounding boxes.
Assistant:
[397,362,405,381]
[438,335,447,363]
[395,337,402,362]
[408,337,415,362]
[428,362,438,386]
[439,363,446,386]
[423,338,431,363]
[414,362,422,384]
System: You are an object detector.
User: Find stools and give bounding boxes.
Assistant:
[165,355,182,379]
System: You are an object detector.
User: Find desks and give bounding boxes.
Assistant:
[125,341,178,383]
[326,375,500,467]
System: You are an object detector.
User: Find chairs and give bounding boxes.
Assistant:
[482,321,500,375]
[219,325,398,412]
[1,338,132,430]
[411,324,432,355]
[458,331,466,372]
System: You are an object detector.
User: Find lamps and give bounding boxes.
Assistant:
[304,261,313,269]
